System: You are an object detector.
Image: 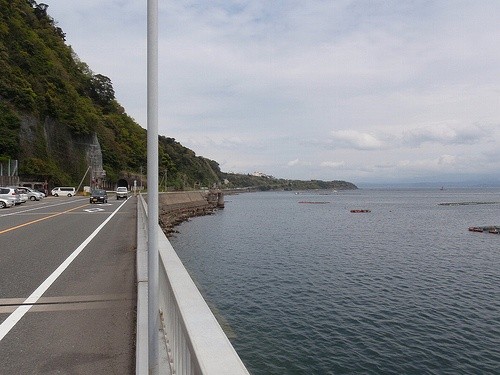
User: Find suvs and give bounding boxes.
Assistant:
[0,187,21,209]
[116,187,127,199]
[17,187,46,201]
[90,189,107,203]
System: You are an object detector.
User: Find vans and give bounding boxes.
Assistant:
[51,187,76,197]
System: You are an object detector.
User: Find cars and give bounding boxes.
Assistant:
[17,189,29,202]
